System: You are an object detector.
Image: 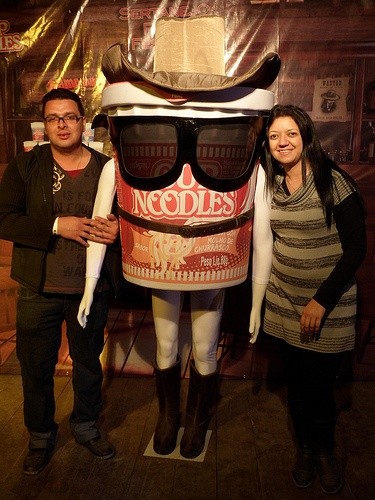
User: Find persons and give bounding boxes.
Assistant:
[0,88,119,475]
[259,104,368,494]
[77,43,281,459]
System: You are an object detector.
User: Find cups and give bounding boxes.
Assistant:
[82,122,104,153]
[101,82,275,290]
[23,121,51,152]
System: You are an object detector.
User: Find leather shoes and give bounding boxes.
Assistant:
[83,437,114,459]
[22,448,54,475]
[292,446,317,487]
[315,454,342,493]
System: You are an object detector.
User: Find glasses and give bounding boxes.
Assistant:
[43,113,83,123]
